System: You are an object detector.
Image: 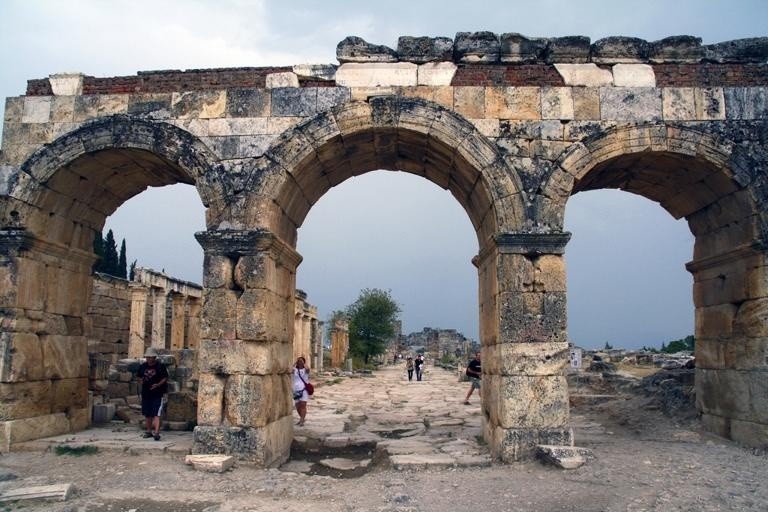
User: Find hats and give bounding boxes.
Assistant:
[143,347,158,358]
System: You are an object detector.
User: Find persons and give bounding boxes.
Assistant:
[133,346,171,443]
[415,355,424,382]
[290,357,313,427]
[391,351,426,364]
[463,351,482,406]
[405,357,414,382]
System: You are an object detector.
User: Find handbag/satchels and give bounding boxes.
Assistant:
[306,383,314,395]
[292,390,303,399]
